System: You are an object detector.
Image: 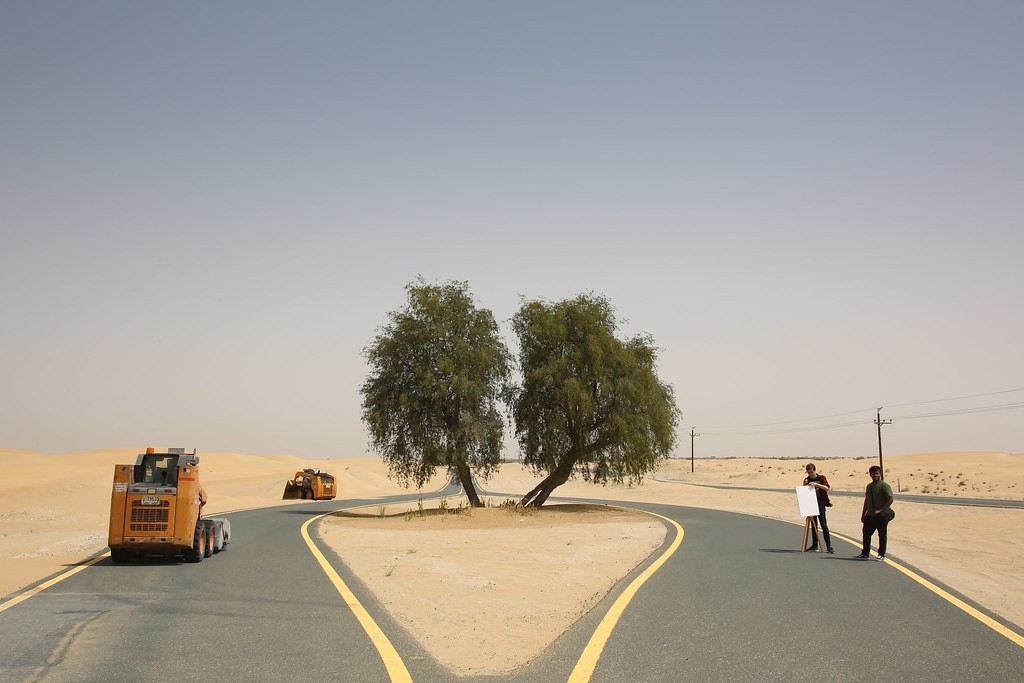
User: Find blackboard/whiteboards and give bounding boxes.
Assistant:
[795,485,820,517]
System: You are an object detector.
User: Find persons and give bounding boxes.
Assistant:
[302,473,316,500]
[803,463,835,554]
[853,466,895,561]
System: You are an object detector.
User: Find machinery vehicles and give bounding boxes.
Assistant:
[108,445,232,563]
[289,467,338,502]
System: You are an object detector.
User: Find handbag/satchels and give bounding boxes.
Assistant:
[876,507,896,524]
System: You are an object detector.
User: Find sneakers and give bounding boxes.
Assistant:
[805,545,820,552]
[874,554,885,561]
[827,545,836,554]
[853,554,868,560]
